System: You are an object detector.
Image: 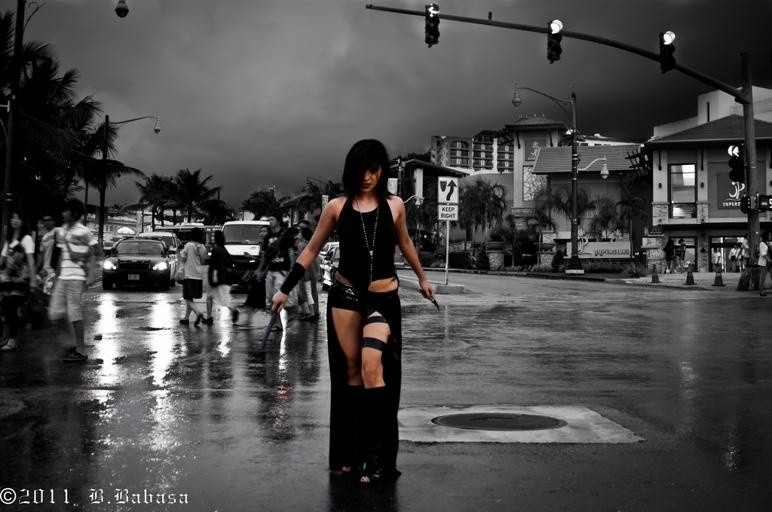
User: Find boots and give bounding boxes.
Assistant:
[341,383,388,483]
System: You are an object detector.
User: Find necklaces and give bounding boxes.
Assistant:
[356,197,379,288]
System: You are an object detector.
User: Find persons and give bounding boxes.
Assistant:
[663,240,679,273]
[202,229,239,325]
[1,205,38,353]
[174,227,208,325]
[43,197,94,362]
[242,225,272,326]
[296,227,319,323]
[38,211,62,279]
[712,232,771,296]
[677,238,687,272]
[272,138,443,487]
[258,210,296,332]
[295,220,322,317]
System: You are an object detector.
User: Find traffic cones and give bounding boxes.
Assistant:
[682,262,698,286]
[711,265,727,287]
[648,263,663,285]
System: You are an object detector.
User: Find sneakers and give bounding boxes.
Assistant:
[232,309,239,321]
[180,313,212,326]
[62,349,89,362]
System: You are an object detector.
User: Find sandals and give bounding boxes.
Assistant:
[3,341,16,350]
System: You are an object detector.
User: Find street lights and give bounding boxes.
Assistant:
[1,1,130,243]
[510,79,609,276]
[403,195,426,203]
[306,175,331,207]
[91,111,164,260]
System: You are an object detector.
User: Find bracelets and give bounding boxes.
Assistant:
[279,262,306,295]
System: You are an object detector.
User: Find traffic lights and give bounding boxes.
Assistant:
[424,3,440,48]
[755,193,772,213]
[658,30,676,75]
[545,18,565,65]
[727,141,747,182]
[740,194,755,213]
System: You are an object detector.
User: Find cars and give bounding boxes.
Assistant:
[104,219,340,290]
